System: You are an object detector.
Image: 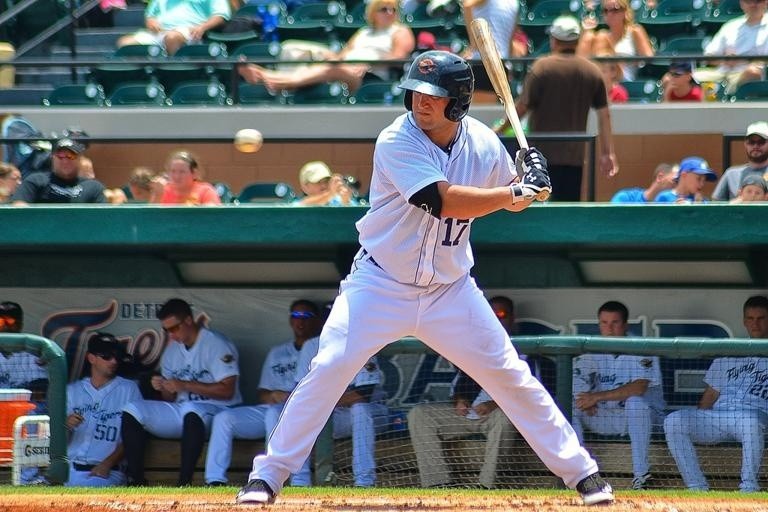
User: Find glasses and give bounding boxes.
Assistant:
[164,322,183,333]
[496,311,506,319]
[95,353,120,361]
[54,153,77,160]
[376,7,397,12]
[601,8,623,13]
[291,311,313,320]
[746,139,766,146]
[0,318,15,326]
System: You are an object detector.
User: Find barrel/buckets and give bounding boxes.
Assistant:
[0,388,35,469]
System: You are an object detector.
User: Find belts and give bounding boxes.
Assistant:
[363,249,382,270]
[72,462,120,472]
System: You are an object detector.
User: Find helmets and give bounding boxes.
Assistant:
[397,51,475,122]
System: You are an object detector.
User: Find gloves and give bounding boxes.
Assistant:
[516,147,548,182]
[511,169,553,204]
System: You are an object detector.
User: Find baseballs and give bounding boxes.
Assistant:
[234,128,261,153]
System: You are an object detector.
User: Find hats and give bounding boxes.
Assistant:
[298,161,332,184]
[0,301,22,318]
[52,138,82,154]
[88,335,133,360]
[666,63,692,72]
[546,17,580,42]
[678,157,717,182]
[746,123,768,139]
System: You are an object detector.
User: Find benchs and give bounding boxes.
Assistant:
[136,432,768,488]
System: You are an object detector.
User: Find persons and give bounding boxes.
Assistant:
[568,301,670,491]
[489,13,620,203]
[660,294,768,490]
[0,300,47,400]
[610,121,768,204]
[285,300,388,487]
[236,49,614,505]
[575,0,768,105]
[203,299,320,486]
[97,0,530,108]
[122,296,243,489]
[1,127,224,207]
[66,332,146,488]
[404,295,547,486]
[291,161,364,207]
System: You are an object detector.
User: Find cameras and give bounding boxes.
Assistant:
[342,176,362,190]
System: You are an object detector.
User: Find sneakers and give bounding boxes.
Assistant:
[236,478,275,504]
[577,471,614,505]
[632,476,648,490]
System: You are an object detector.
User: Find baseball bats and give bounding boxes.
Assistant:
[469,18,552,203]
